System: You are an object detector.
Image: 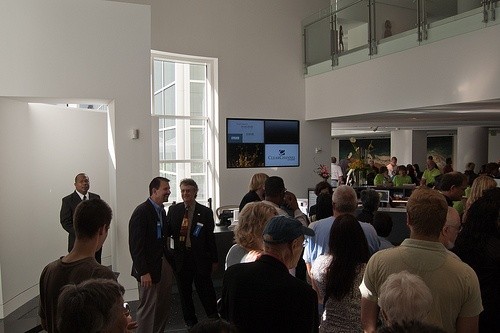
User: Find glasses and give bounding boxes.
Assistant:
[295,238,308,248]
[281,187,288,192]
[447,224,463,233]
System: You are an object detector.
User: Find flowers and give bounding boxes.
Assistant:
[317,165,331,178]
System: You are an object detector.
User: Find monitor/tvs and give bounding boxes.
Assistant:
[225,118,300,167]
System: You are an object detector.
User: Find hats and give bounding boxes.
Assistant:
[262,217,315,244]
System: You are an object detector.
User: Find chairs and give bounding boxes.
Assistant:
[216,206,240,224]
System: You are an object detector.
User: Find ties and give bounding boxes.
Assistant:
[185,208,188,236]
[83,195,87,201]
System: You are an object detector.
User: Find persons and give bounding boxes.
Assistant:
[331,155,500,188]
[221,172,500,333]
[129,177,171,333]
[358,188,483,333]
[38,199,118,333]
[310,214,373,333]
[61,173,102,264]
[378,270,449,333]
[163,179,220,333]
[190,317,237,333]
[220,215,320,333]
[55,277,138,333]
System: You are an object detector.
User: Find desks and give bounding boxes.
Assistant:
[213,225,241,252]
